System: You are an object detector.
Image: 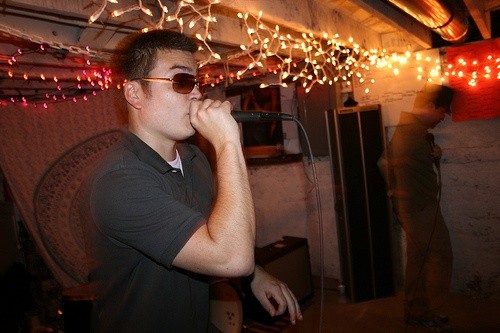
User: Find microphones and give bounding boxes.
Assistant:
[426,133,440,170]
[231,109,294,122]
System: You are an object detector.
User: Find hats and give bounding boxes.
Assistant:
[416,82,456,116]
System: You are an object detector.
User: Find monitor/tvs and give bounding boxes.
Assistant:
[239,235,315,325]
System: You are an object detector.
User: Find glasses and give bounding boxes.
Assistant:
[130,73,199,94]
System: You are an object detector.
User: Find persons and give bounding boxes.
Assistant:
[385,83,456,333]
[88,30,304,333]
